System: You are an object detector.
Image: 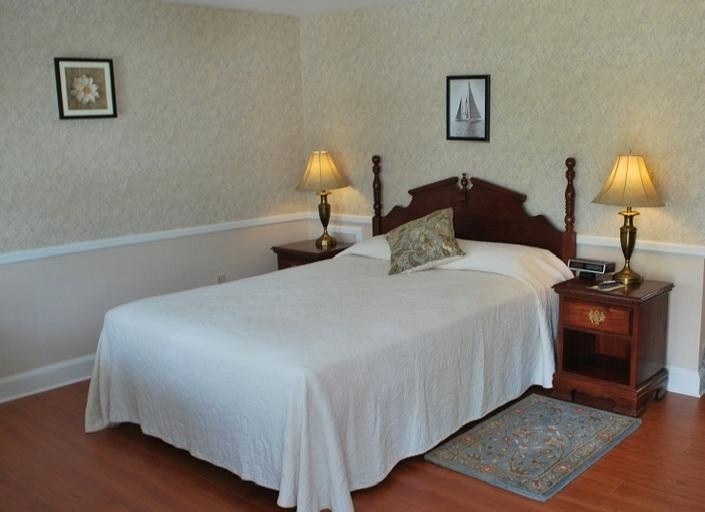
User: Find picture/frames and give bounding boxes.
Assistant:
[54,56,117,119]
[447,74,490,141]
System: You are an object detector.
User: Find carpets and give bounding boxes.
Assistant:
[424,392,643,503]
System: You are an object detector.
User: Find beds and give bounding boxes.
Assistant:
[84,155,576,512]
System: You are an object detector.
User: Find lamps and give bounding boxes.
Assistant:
[590,152,665,286]
[296,150,350,247]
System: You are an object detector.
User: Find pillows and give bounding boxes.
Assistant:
[384,206,466,275]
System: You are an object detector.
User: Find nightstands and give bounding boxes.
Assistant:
[272,239,352,271]
[550,274,675,417]
